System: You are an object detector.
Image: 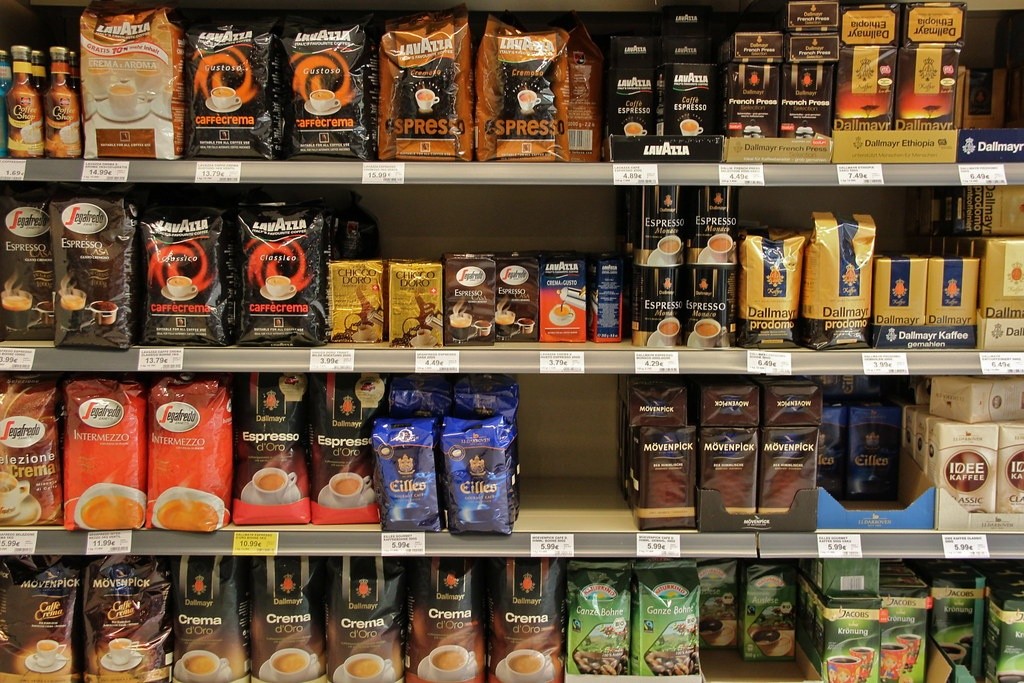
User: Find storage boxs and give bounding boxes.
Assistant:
[322,249,624,348]
[609,0,1023,161]
[873,183,1023,351]
[610,374,1024,536]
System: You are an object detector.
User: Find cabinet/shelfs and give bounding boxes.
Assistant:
[1,160,1024,560]
